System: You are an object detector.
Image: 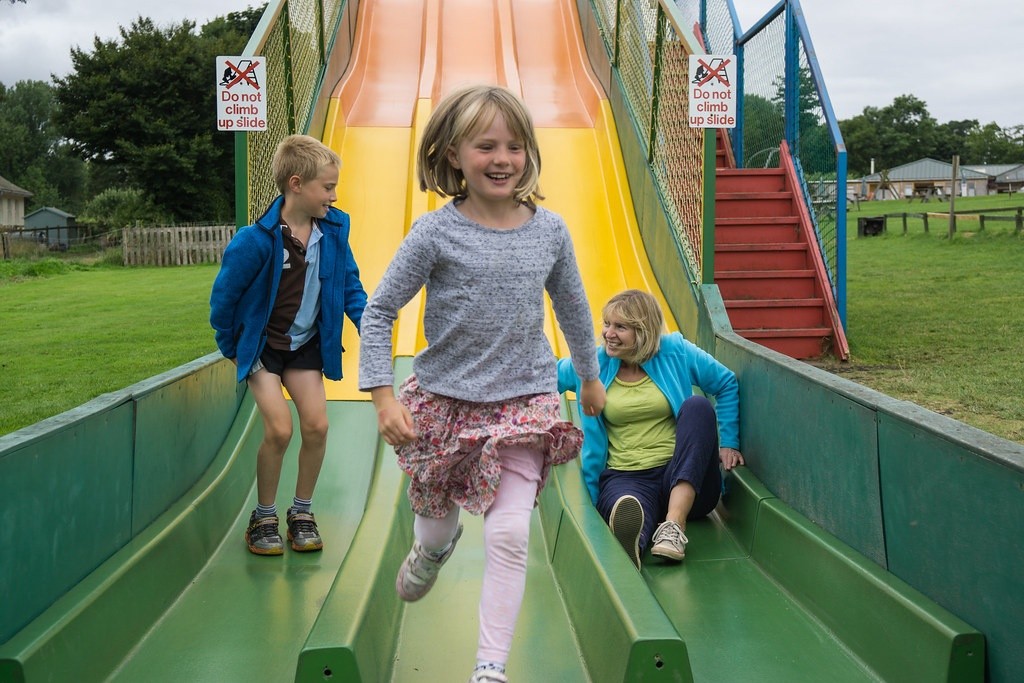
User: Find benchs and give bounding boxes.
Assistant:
[812,200,850,222]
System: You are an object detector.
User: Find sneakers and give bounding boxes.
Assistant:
[245,509,284,554]
[396,520,464,602]
[286,505,322,550]
[469,665,507,683]
[650,522,688,560]
[609,494,644,573]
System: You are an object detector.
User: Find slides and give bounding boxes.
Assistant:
[0,0,987,683]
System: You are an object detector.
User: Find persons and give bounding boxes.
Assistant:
[208,136,369,555]
[361,86,608,682]
[555,290,744,572]
